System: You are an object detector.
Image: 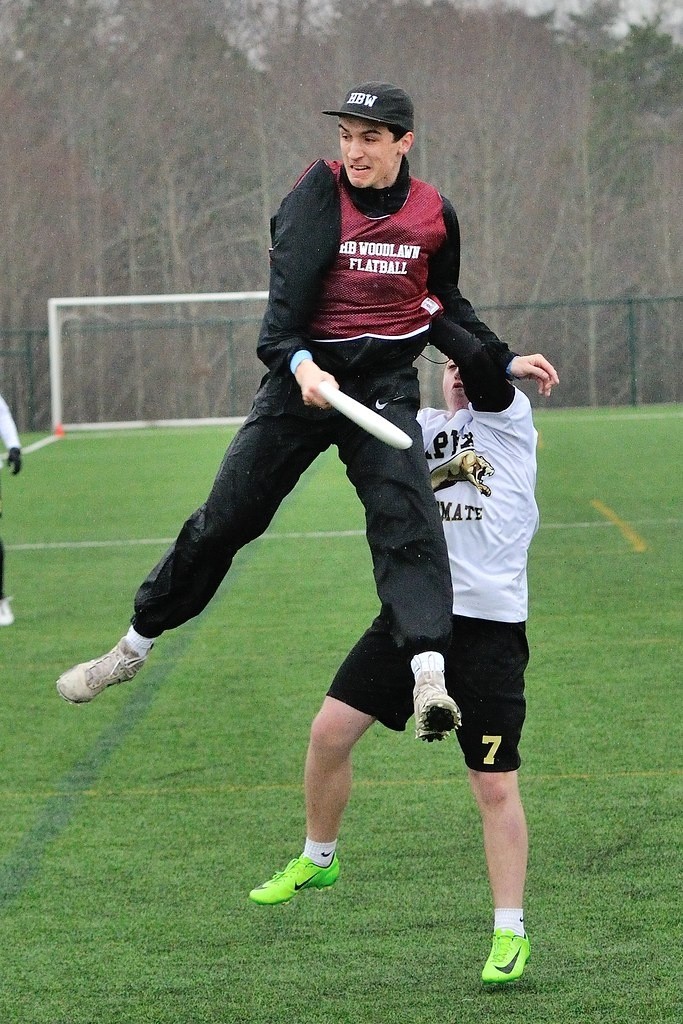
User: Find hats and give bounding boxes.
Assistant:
[322,81,415,131]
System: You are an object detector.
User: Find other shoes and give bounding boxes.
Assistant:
[0,597,15,625]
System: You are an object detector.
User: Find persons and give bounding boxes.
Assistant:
[249,358,541,984]
[55,83,560,742]
[0,394,22,626]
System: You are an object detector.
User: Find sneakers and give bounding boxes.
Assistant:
[481,926,531,986]
[412,654,462,744]
[57,636,155,707]
[249,852,340,905]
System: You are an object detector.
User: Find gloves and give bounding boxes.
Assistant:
[7,447,22,474]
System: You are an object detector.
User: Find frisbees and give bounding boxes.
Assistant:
[318,379,414,452]
